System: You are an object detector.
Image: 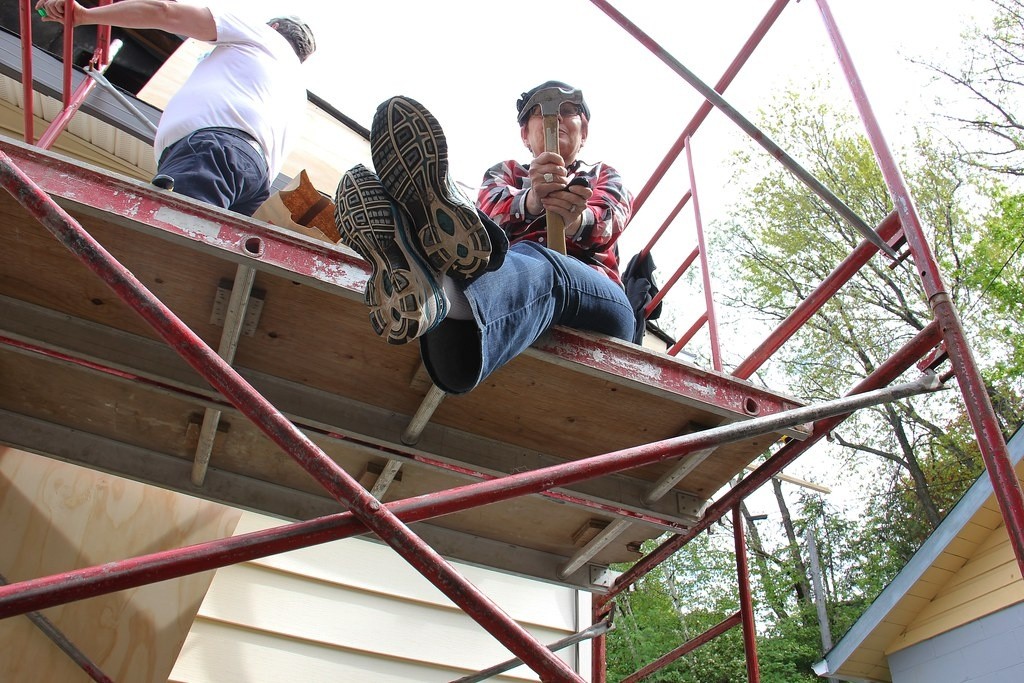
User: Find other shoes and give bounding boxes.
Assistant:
[333,164,451,346]
[370,95,492,280]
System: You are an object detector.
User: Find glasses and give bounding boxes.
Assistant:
[530,106,580,118]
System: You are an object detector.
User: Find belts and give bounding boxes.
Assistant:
[200,127,267,171]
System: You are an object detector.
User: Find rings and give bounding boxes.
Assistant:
[544,173,554,183]
[571,204,576,213]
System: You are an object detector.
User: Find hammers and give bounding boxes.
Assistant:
[517,87,584,257]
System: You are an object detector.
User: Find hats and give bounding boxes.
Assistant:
[265,15,317,55]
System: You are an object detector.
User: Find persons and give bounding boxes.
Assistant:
[334,80,637,396]
[34,0,317,216]
